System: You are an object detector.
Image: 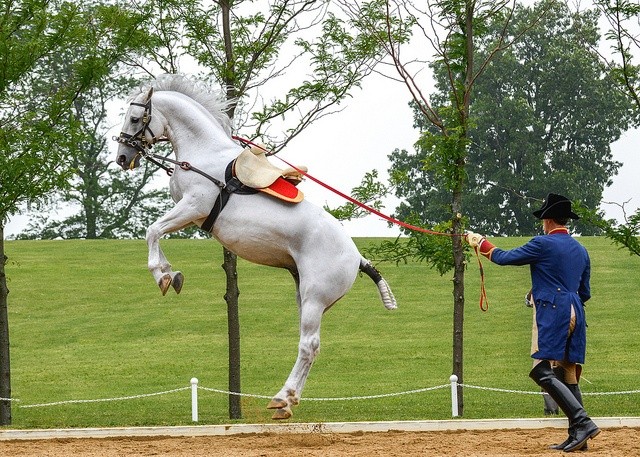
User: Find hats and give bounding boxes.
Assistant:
[532,192,579,220]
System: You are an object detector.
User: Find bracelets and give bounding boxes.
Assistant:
[478,237,487,244]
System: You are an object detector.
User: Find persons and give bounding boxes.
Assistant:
[463,194,601,451]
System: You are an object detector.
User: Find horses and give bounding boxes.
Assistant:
[116,75,396,419]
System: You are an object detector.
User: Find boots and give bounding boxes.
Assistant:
[529,360,601,452]
[549,365,587,451]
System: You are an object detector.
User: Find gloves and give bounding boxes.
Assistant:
[465,233,485,247]
